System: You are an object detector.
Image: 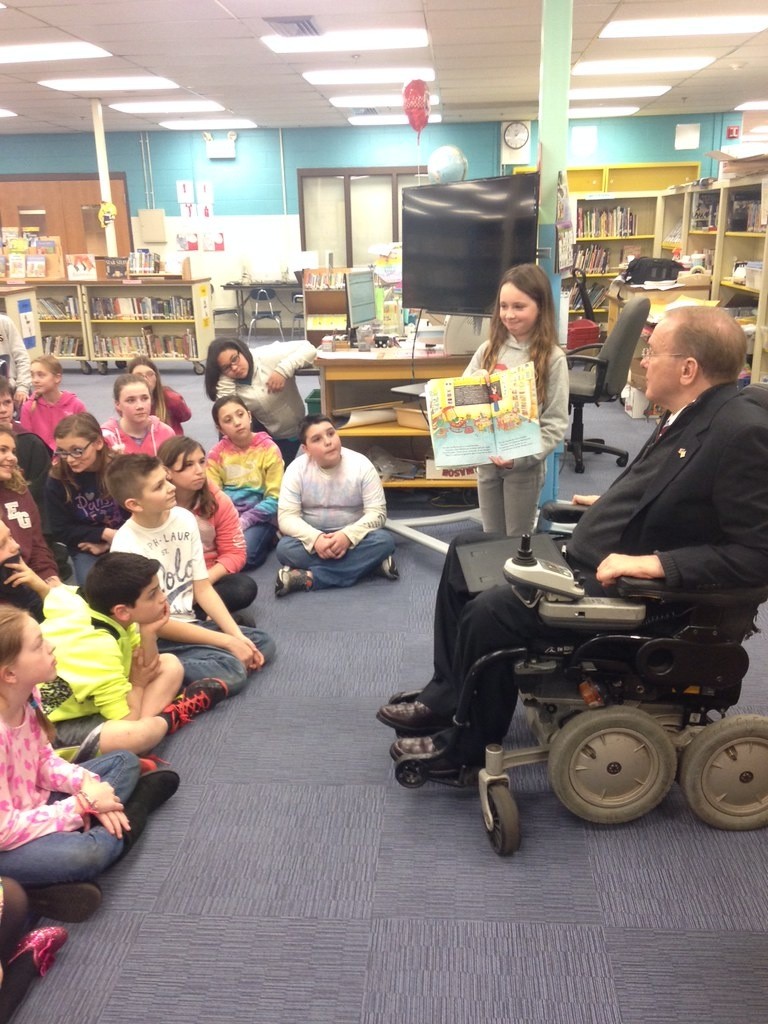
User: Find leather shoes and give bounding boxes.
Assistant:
[389,735,438,762]
[376,700,450,735]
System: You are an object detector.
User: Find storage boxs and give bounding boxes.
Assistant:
[624,383,667,419]
[630,357,649,392]
[391,398,430,431]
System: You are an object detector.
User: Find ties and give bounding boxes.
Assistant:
[651,420,671,444]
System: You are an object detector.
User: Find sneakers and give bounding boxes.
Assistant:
[370,555,398,578]
[274,565,311,597]
[138,754,164,775]
[161,678,228,735]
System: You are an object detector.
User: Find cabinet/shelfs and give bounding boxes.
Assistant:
[0,277,215,375]
[568,173,768,386]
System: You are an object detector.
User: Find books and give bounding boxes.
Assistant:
[619,245,642,268]
[93,326,197,358]
[66,254,127,279]
[0,226,54,278]
[128,249,160,273]
[36,295,80,319]
[42,334,84,356]
[424,362,543,470]
[696,192,767,233]
[90,296,194,320]
[573,246,611,309]
[571,207,637,239]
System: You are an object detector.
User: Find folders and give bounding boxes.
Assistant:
[65,296,79,319]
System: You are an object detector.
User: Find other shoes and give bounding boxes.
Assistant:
[4,927,67,978]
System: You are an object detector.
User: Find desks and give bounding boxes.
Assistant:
[314,337,480,508]
[220,280,303,340]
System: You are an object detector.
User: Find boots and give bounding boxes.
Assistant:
[20,880,102,924]
[118,769,180,861]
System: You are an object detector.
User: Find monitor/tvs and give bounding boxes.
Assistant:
[402,172,540,318]
[343,269,377,347]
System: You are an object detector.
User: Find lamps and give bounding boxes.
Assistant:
[202,130,237,158]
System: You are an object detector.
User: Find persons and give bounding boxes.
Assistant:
[0,603,180,1024]
[462,265,570,536]
[206,396,284,568]
[205,337,317,469]
[377,306,768,779]
[0,356,276,772]
[0,315,33,419]
[275,414,401,596]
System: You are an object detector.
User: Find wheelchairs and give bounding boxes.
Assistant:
[391,500,768,857]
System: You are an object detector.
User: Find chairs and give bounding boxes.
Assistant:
[564,296,651,474]
[210,284,238,317]
[248,288,285,347]
[290,290,305,341]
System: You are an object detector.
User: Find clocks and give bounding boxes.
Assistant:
[504,122,529,149]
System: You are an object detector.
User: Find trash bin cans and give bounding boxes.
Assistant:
[304,389,321,416]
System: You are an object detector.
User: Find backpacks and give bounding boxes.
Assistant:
[622,256,684,284]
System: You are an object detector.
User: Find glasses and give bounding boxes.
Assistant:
[220,351,240,374]
[55,440,93,459]
[136,373,155,378]
[642,347,682,362]
